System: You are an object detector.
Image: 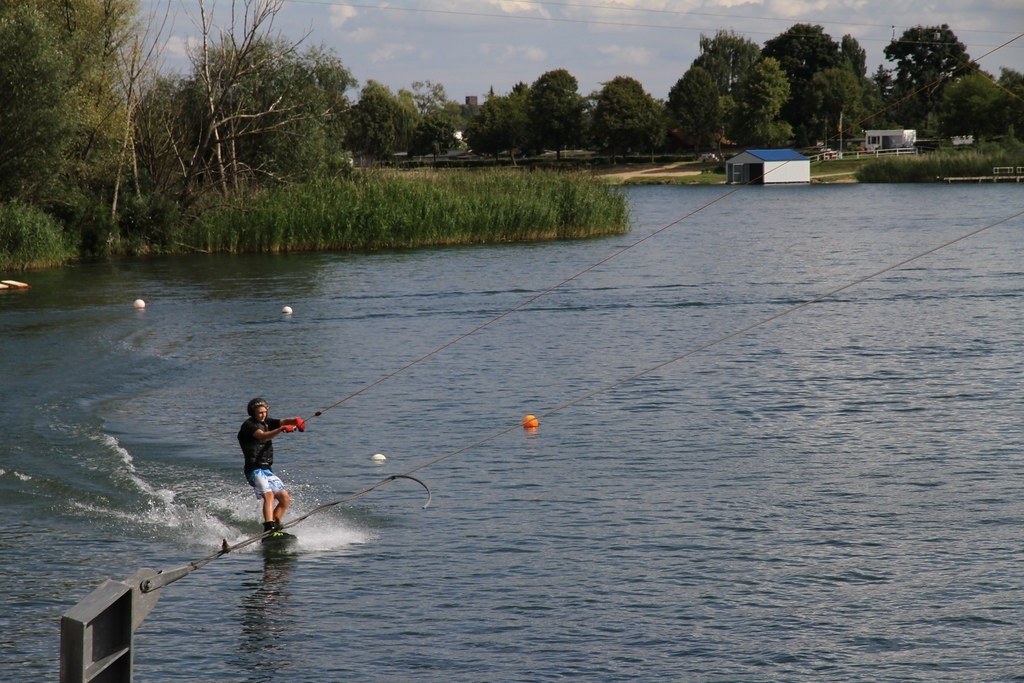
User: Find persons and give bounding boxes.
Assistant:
[237,398,305,540]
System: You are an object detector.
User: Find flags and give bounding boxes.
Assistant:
[838,113,842,132]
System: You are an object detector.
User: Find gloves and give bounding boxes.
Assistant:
[294,417,305,433]
[279,424,293,433]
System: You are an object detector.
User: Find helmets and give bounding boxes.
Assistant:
[247,397,269,417]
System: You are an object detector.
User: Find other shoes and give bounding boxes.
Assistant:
[263,521,289,539]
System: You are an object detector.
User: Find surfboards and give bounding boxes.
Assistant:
[262,531,298,544]
